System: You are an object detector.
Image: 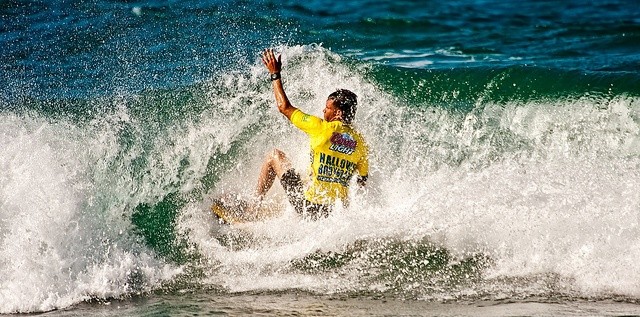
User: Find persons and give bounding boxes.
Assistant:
[249,48,370,222]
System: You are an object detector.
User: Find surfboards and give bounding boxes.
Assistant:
[212,196,287,236]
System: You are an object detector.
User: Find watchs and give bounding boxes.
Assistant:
[271,73,281,81]
